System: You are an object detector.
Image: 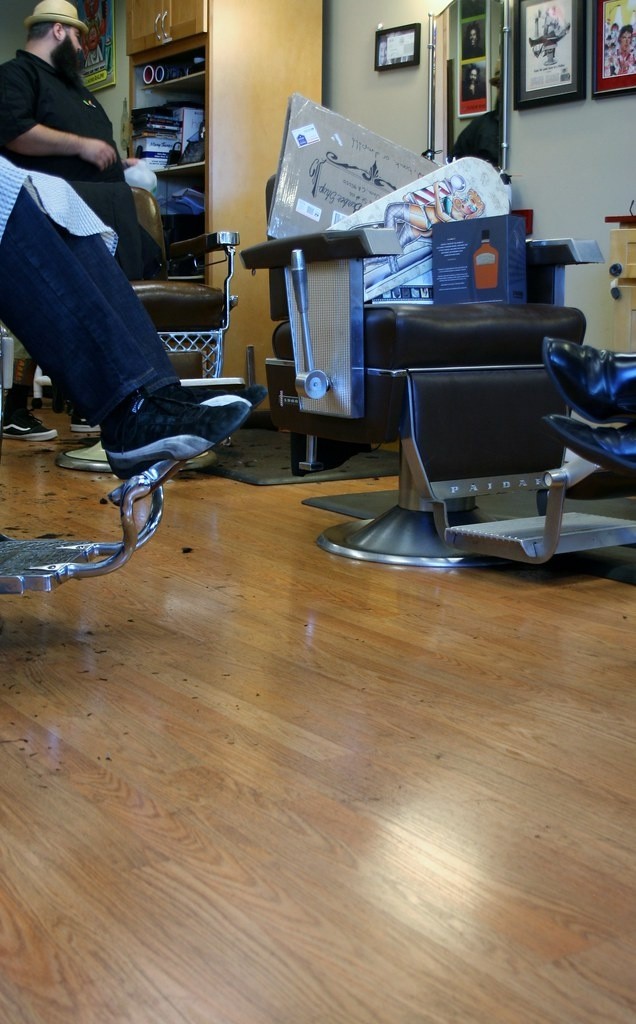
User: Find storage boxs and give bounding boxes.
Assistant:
[264,92,529,305]
[130,107,204,170]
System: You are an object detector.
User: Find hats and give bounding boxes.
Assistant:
[23,0,89,36]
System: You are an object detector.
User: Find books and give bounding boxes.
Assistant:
[131,114,183,140]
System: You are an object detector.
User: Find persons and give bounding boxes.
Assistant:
[609,25,636,77]
[539,335,636,479]
[0,0,144,440]
[461,22,486,60]
[605,24,618,51]
[463,62,485,101]
[0,153,266,482]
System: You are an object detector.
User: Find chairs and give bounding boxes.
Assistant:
[238,173,636,567]
[127,184,247,389]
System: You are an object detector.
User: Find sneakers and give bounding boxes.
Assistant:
[70,410,101,432]
[2,409,58,441]
[100,383,268,479]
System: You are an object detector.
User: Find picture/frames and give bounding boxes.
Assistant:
[512,0,587,110]
[590,0,636,101]
[374,22,420,71]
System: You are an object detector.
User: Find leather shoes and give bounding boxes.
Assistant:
[539,335,636,477]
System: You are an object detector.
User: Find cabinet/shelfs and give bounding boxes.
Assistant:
[126,0,325,414]
